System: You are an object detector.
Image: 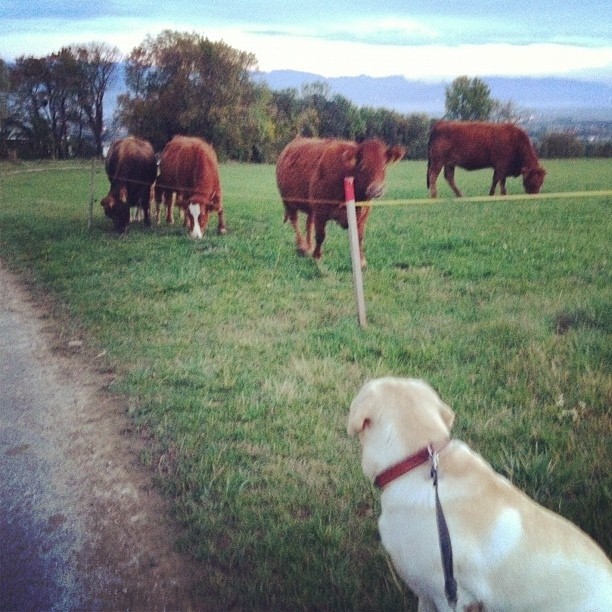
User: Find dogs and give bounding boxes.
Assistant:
[347,376,612,612]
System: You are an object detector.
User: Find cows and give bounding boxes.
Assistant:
[427,121,548,198]
[275,136,405,270]
[153,134,227,238]
[99,136,158,235]
